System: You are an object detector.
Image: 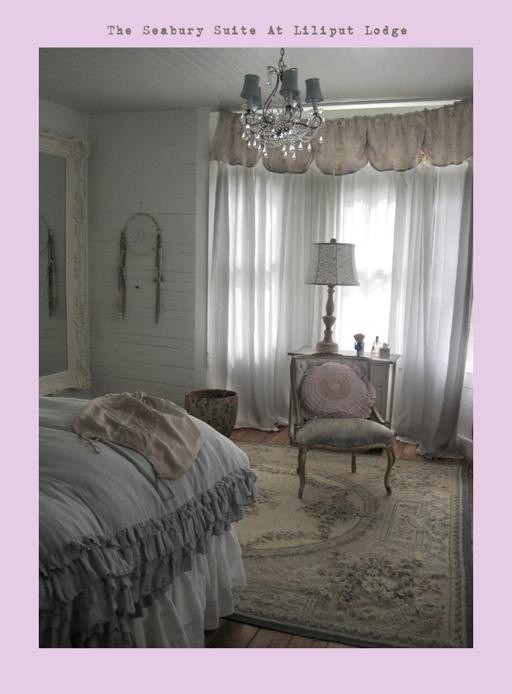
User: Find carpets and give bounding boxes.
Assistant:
[219,440,473,648]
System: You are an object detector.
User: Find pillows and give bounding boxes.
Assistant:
[299,361,376,420]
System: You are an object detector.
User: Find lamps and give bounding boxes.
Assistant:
[239,47,323,161]
[304,239,360,353]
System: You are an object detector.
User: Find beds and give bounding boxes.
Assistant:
[39,393,257,648]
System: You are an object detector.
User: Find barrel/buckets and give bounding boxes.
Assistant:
[184,388,239,438]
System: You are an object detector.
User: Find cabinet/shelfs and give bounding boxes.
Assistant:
[287,345,400,455]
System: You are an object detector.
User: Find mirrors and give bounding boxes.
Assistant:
[39,128,91,397]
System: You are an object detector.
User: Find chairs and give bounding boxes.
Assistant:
[290,353,396,498]
